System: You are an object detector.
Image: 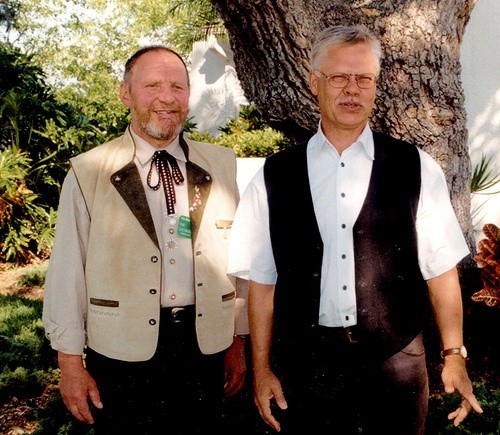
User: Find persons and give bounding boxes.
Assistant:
[222,25,486,435]
[40,46,253,434]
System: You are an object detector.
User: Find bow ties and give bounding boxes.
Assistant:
[147,150,184,215]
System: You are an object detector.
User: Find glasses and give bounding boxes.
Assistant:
[317,69,378,89]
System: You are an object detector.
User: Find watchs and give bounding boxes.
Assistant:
[440,346,469,360]
